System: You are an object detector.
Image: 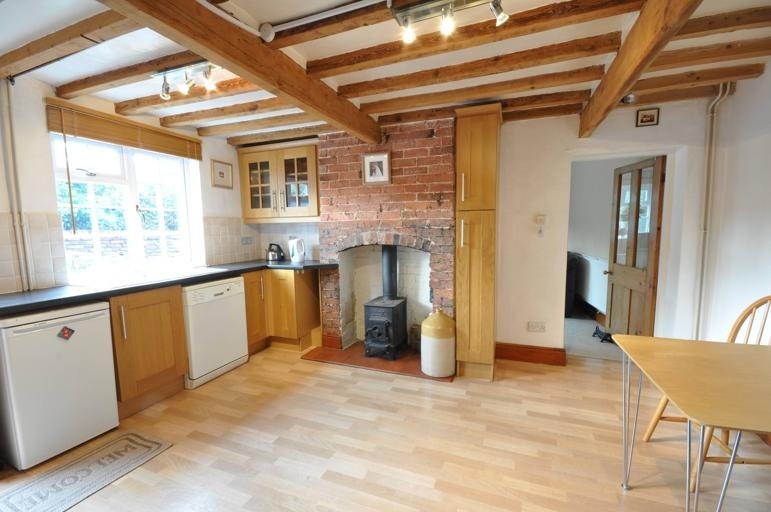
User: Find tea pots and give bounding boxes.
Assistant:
[265,243,284,262]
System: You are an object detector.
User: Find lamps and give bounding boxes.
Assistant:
[489,0,508,28]
[158,72,172,101]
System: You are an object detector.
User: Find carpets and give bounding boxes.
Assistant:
[0,428,175,511]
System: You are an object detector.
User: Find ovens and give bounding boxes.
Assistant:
[182,277,251,391]
[1,302,126,470]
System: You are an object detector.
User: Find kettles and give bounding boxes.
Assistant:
[288,236,305,263]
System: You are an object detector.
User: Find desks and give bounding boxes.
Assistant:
[607,333,771,511]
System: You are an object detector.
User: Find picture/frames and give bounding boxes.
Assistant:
[210,158,233,190]
[635,108,659,126]
[360,150,391,185]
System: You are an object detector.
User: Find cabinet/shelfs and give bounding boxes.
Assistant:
[240,268,267,345]
[265,268,318,339]
[452,111,500,364]
[109,284,187,405]
[239,146,319,219]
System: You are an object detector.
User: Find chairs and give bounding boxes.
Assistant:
[641,294,771,493]
